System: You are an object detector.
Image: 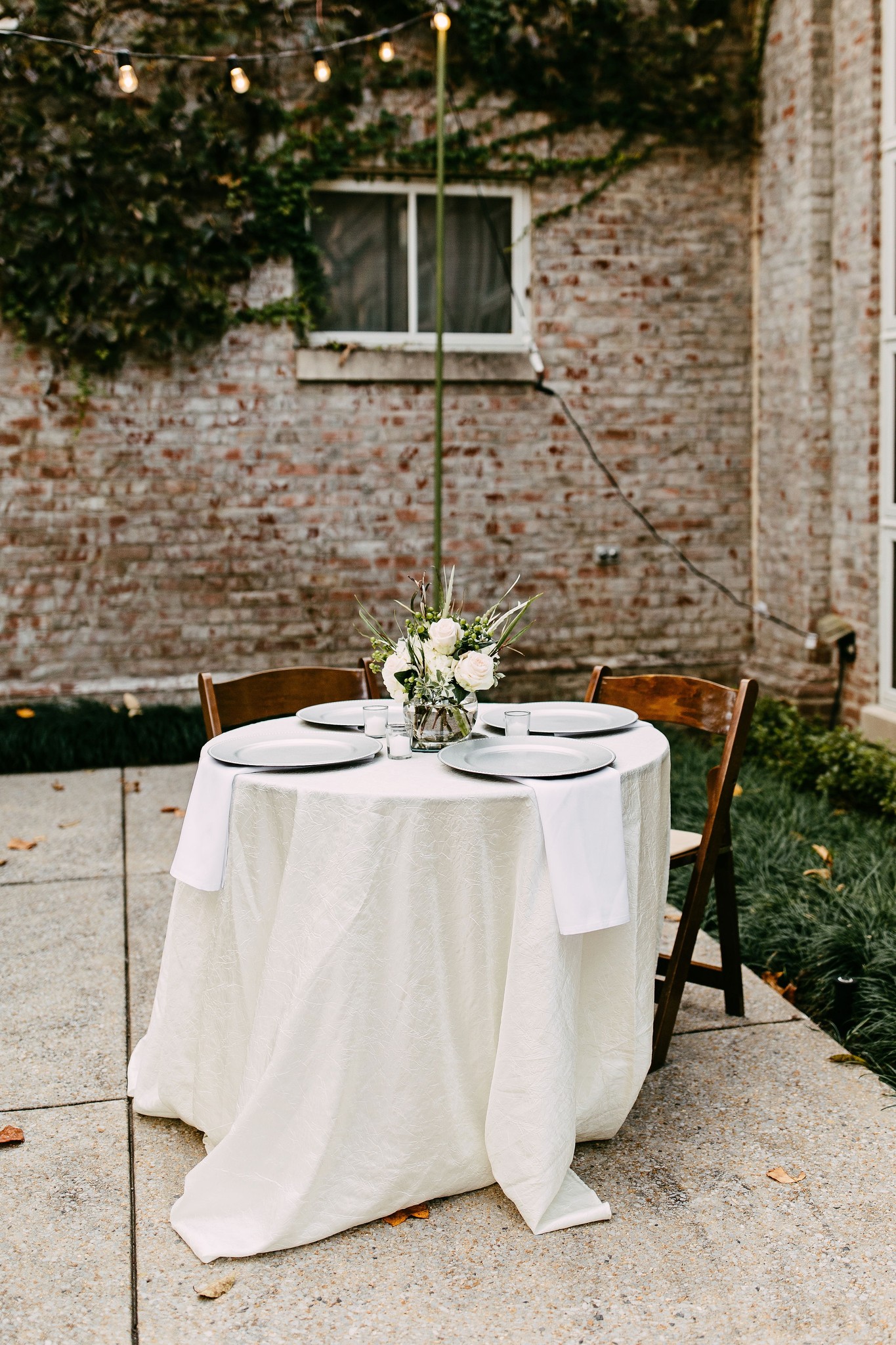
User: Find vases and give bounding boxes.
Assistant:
[402,689,478,752]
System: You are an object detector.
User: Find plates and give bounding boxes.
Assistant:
[296,697,416,727]
[206,728,383,770]
[479,698,639,733]
[437,734,617,779]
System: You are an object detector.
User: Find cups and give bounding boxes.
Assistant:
[362,704,389,739]
[385,722,414,760]
[504,711,530,737]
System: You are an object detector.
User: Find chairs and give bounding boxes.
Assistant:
[583,664,760,1075]
[196,655,379,747]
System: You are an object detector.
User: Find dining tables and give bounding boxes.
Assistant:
[122,701,674,1260]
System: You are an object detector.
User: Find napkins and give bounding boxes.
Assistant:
[502,764,635,941]
[169,749,283,894]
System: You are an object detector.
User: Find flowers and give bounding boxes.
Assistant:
[352,565,543,742]
